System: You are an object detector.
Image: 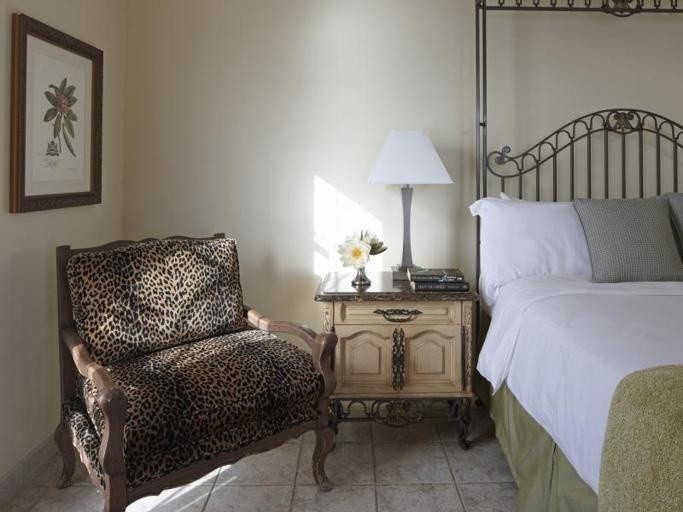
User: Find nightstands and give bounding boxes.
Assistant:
[312,268,479,450]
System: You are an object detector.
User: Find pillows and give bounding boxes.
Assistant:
[469,191,682,291]
[64,237,248,367]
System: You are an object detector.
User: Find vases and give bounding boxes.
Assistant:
[351,268,371,285]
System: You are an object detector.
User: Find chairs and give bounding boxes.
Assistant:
[54,231,339,512]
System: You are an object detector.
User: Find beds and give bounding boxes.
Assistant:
[466,1,682,512]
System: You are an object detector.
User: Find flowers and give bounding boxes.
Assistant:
[336,229,388,270]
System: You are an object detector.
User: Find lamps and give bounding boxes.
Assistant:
[365,128,455,279]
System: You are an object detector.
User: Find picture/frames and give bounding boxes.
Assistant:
[8,12,102,213]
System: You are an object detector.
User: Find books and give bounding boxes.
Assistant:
[406,267,465,283]
[410,280,470,292]
